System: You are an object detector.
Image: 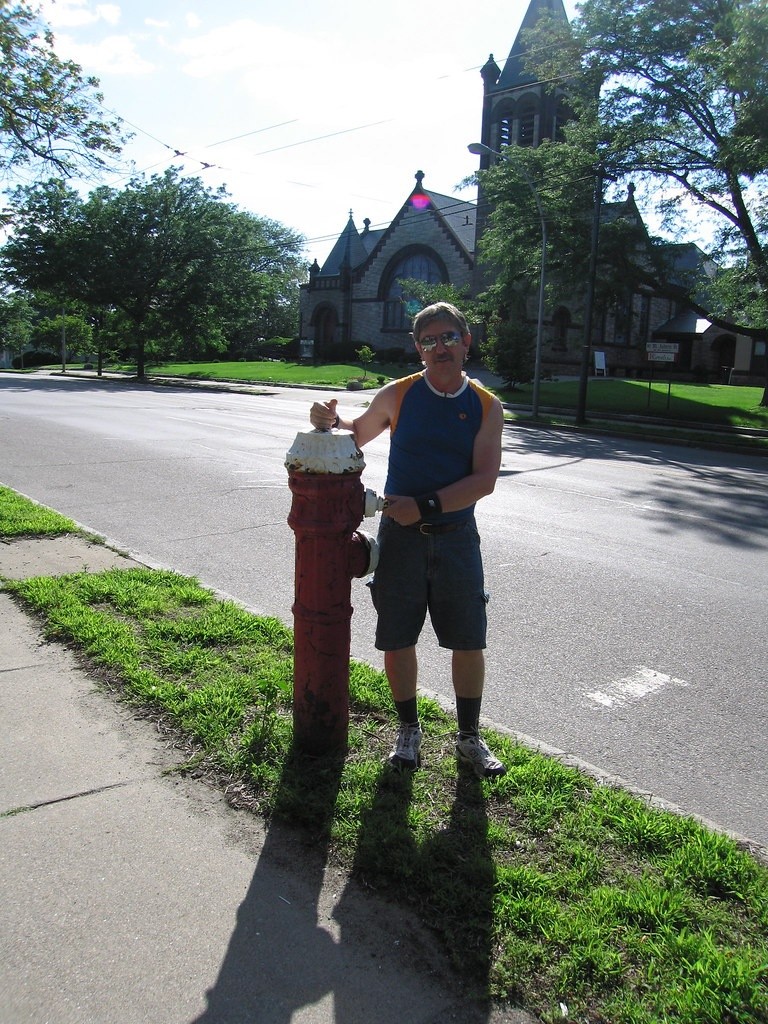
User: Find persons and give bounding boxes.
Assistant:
[310,302,507,774]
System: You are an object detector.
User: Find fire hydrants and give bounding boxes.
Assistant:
[284,407,395,751]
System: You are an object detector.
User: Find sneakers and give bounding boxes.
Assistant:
[454,732,506,780]
[389,725,423,772]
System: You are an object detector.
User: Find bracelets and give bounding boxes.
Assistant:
[333,414,339,428]
[414,492,443,519]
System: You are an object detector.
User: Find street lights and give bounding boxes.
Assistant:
[467,141,545,416]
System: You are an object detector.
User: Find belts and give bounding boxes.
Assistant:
[382,513,470,535]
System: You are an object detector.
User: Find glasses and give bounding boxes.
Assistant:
[418,331,464,352]
